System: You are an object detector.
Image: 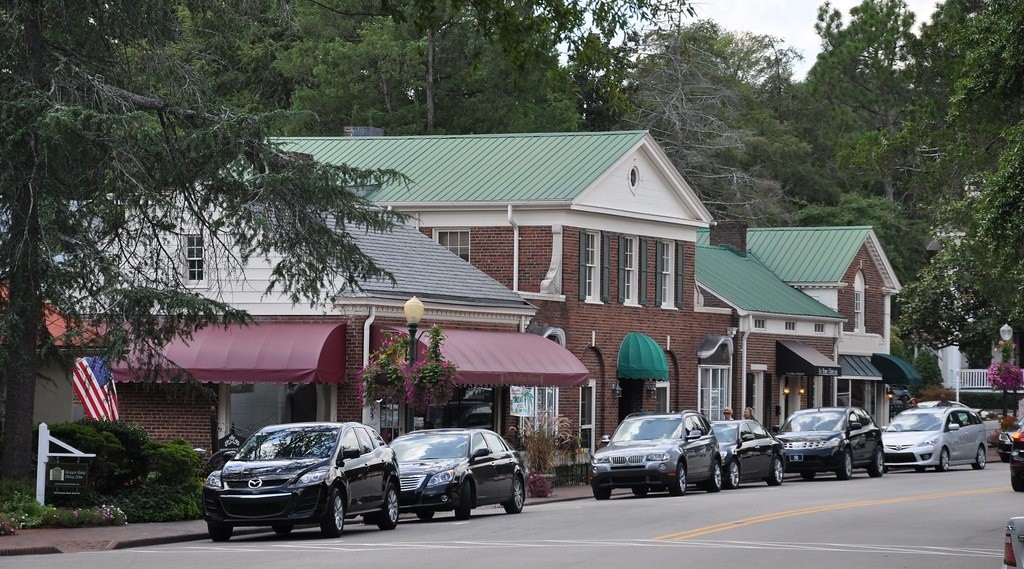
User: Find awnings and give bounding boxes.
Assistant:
[617,333,669,382]
[776,340,842,377]
[93,318,348,384]
[838,355,883,380]
[871,353,923,384]
[369,324,590,388]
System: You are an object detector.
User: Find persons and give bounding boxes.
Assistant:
[937,394,951,406]
[743,407,759,422]
[723,407,734,420]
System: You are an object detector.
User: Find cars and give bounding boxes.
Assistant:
[363,428,526,526]
[916,401,1002,448]
[880,406,988,472]
[1010,424,1024,492]
[1003,516,1024,569]
[997,416,1024,464]
[649,419,785,490]
[202,421,401,543]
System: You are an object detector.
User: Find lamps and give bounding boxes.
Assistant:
[611,380,622,398]
[646,381,657,400]
[886,387,895,402]
[798,385,806,394]
[782,387,790,395]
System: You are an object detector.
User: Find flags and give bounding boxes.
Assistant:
[72,354,119,422]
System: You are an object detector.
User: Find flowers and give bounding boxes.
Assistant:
[986,340,1024,392]
[358,326,458,422]
[986,414,1020,446]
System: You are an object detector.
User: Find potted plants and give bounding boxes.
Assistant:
[507,407,581,498]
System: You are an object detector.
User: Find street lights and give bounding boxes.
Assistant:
[403,295,425,435]
[1000,323,1013,419]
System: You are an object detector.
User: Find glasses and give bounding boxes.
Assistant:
[724,413,729,415]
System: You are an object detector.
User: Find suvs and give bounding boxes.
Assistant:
[772,406,885,481]
[590,409,723,501]
